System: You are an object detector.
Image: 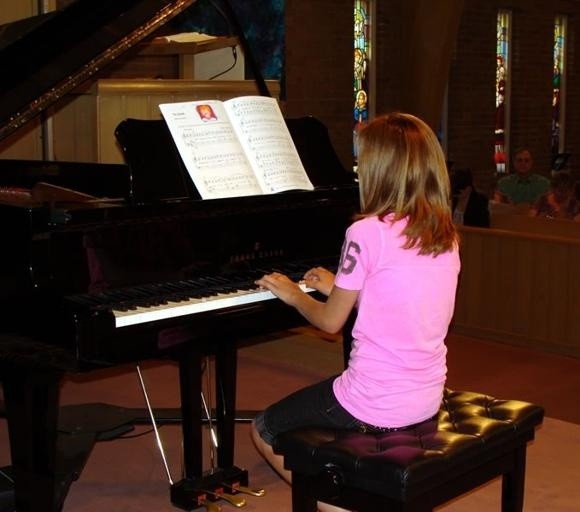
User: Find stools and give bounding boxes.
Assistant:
[271,385,545,512]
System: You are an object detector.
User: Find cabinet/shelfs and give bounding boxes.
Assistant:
[98,29,246,80]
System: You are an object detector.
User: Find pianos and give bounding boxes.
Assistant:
[0,159,363,512]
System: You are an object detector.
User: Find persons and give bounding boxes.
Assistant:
[250,112,463,512]
[528,169,580,222]
[450,169,490,229]
[497,146,550,204]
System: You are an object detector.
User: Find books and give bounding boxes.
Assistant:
[158,94,318,200]
